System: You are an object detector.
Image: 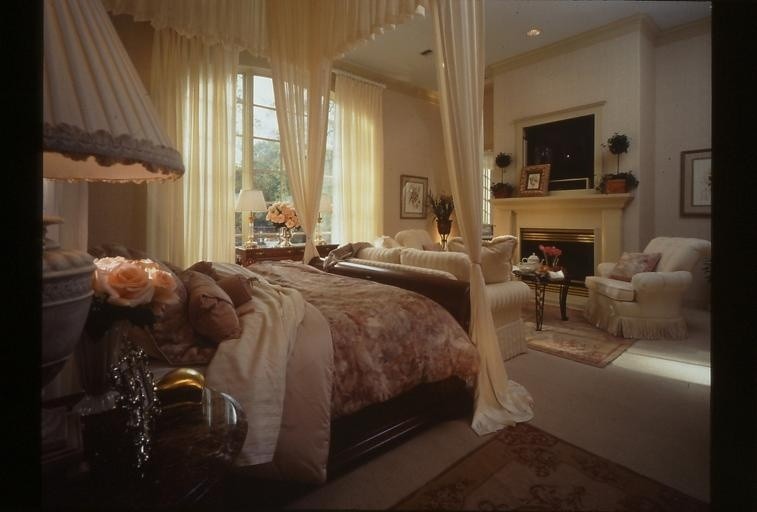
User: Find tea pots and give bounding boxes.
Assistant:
[521,252,545,266]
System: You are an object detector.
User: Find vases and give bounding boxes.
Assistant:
[283,228,292,247]
[436,220,452,234]
[541,264,561,272]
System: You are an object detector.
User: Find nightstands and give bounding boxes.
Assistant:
[40,387,248,504]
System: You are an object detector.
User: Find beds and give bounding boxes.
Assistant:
[86,258,479,504]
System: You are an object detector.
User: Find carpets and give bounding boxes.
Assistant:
[521,301,637,368]
[386,422,709,512]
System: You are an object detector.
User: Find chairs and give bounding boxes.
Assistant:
[583,236,711,341]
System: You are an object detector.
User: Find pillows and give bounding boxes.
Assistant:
[87,242,260,367]
[609,251,661,281]
[356,230,518,287]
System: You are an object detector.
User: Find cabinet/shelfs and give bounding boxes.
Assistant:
[235,244,339,266]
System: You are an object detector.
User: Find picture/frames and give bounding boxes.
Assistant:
[680,149,711,218]
[400,175,428,219]
[520,164,551,197]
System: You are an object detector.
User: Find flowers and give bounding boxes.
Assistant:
[266,201,301,231]
[539,245,562,266]
[428,190,454,220]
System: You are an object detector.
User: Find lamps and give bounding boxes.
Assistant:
[236,189,267,245]
[41,0,185,399]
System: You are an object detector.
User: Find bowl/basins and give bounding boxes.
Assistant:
[518,263,541,273]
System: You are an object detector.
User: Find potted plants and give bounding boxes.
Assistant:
[490,152,513,198]
[595,132,639,193]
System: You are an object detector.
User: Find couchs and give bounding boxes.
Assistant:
[331,258,530,362]
